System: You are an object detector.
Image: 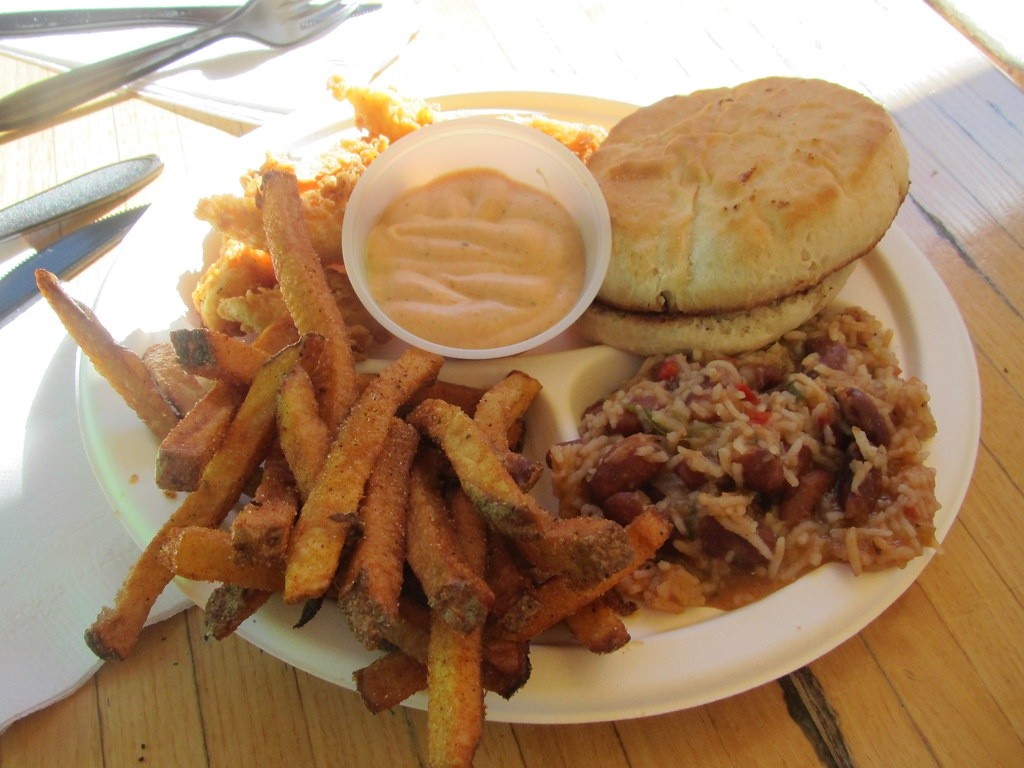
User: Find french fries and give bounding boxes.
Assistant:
[31,173,671,768]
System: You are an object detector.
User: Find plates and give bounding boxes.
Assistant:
[76,63,983,722]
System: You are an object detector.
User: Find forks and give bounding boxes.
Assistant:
[0,1,363,139]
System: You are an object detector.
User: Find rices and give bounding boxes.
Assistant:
[546,306,946,610]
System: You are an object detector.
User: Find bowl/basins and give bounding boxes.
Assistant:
[341,117,611,361]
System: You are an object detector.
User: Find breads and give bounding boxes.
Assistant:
[569,78,911,354]
